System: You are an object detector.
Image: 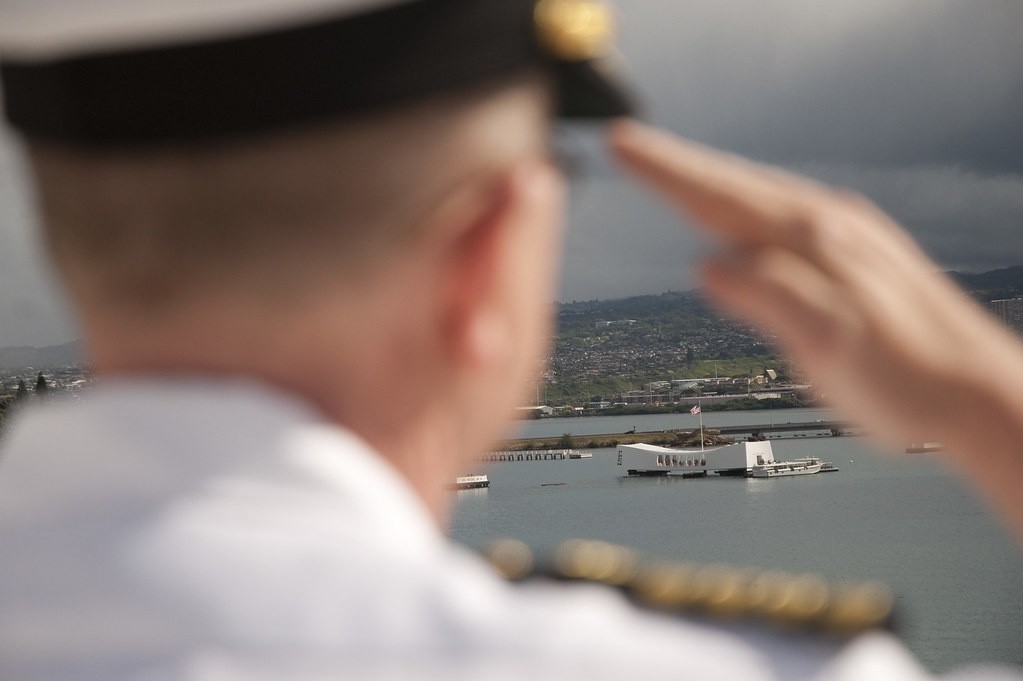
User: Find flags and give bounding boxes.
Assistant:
[690,405,701,416]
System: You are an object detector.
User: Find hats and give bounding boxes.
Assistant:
[0,0,630,117]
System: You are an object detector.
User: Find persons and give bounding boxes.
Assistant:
[1,0,1023,681]
